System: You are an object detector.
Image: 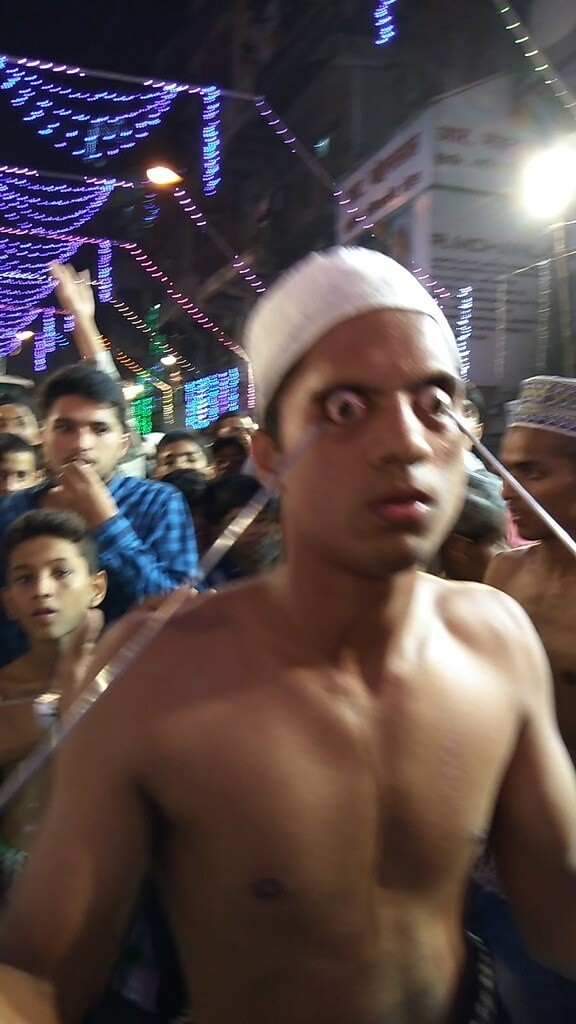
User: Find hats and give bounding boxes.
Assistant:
[503,375,576,437]
[243,247,461,432]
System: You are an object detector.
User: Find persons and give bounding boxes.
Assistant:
[0,245,576,1024]
